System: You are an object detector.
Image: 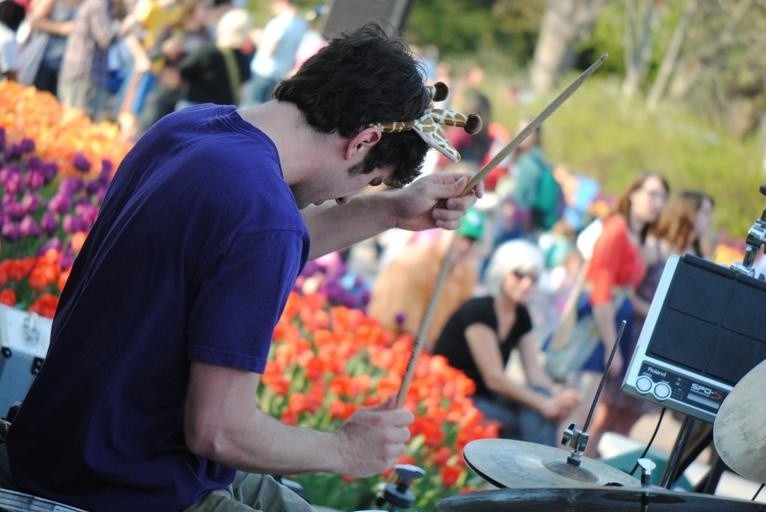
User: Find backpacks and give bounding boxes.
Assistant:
[526,153,564,230]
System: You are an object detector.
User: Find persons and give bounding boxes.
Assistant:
[1,0,715,487]
[0,21,488,512]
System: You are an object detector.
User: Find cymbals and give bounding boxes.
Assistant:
[463,439,645,489]
[714,358,766,482]
[439,487,766,512]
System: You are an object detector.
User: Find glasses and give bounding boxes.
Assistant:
[515,270,536,281]
